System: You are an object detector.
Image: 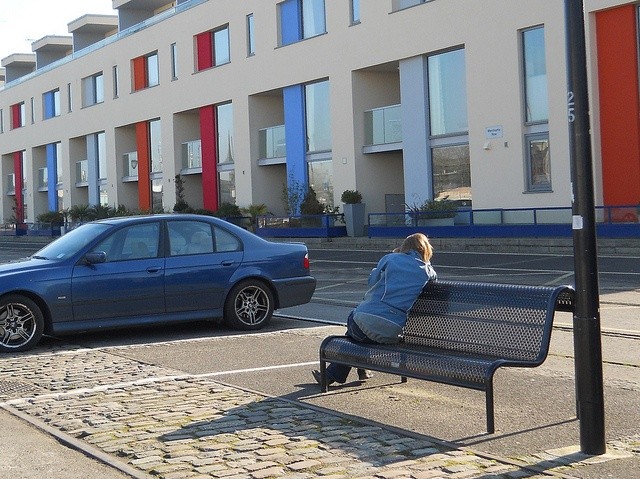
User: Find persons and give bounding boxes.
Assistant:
[312,232,437,387]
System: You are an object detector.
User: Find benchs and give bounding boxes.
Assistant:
[318,274,580,434]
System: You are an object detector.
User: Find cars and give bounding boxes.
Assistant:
[0,215,316,351]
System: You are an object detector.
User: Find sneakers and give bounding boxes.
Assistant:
[358,368,374,380]
[312,368,334,386]
